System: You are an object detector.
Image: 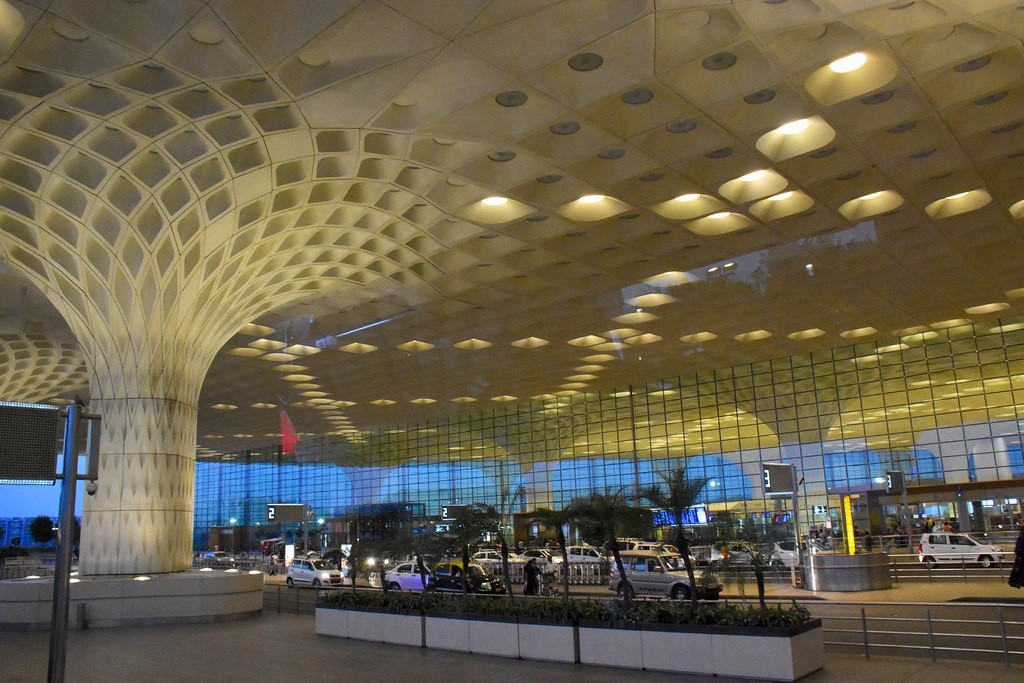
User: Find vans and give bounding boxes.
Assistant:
[710,541,768,563]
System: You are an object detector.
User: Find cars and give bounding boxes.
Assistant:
[260,537,283,556]
[769,539,842,566]
[469,550,520,565]
[205,551,235,562]
[565,536,697,565]
[383,562,432,592]
[284,557,345,588]
[516,548,565,564]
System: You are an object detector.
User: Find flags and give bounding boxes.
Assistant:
[279,405,297,453]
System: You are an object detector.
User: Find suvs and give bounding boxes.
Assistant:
[917,532,1005,569]
[609,550,724,601]
[427,558,507,595]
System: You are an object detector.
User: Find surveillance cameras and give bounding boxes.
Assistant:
[86,483,97,495]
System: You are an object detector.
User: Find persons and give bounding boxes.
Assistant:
[818,528,826,545]
[721,544,731,564]
[521,557,543,595]
[516,535,558,550]
[214,544,225,551]
[269,552,278,576]
[860,531,873,552]
[853,524,859,537]
[894,520,905,535]
[922,517,957,532]
